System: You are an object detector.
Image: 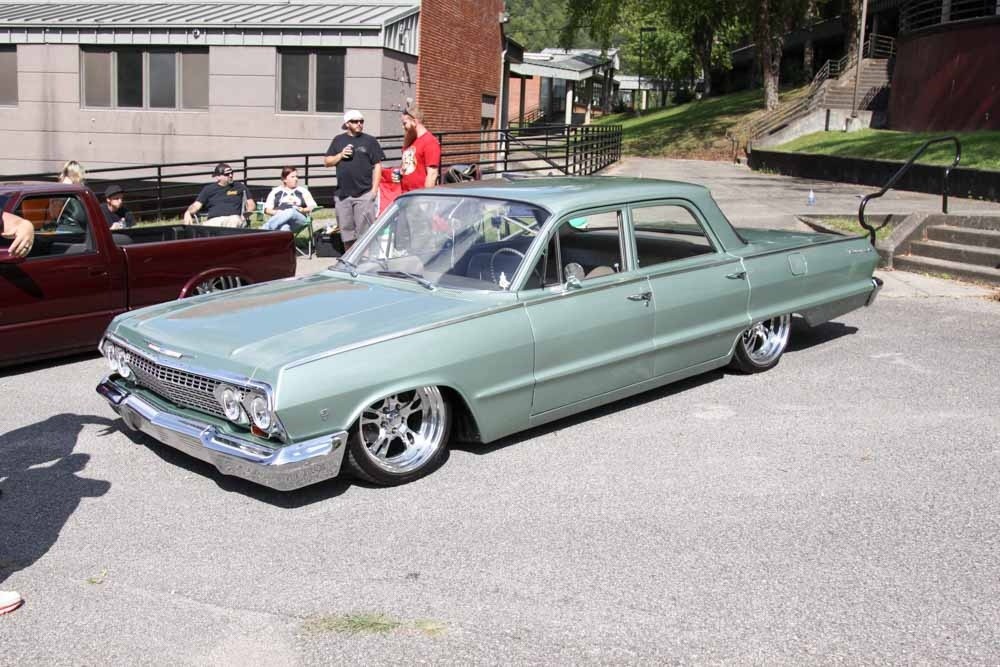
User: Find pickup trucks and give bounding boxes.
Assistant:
[0,178,299,371]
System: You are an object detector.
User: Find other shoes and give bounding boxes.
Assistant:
[0,590,22,615]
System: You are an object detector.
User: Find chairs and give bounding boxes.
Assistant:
[195,188,252,229]
[252,186,324,260]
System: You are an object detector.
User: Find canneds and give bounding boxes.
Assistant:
[394,168,400,182]
[346,144,354,156]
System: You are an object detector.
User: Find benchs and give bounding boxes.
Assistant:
[463,252,614,298]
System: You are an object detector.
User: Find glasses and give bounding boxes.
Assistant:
[223,172,232,176]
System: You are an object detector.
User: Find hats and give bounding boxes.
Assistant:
[342,110,365,129]
[104,186,122,198]
[212,164,232,177]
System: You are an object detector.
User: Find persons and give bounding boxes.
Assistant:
[322,110,385,261]
[391,107,439,256]
[1,211,34,256]
[99,184,138,229]
[49,160,86,234]
[185,164,256,228]
[262,167,317,232]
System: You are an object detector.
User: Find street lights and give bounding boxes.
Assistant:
[636,27,658,116]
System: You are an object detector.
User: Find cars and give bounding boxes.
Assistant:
[94,178,886,493]
